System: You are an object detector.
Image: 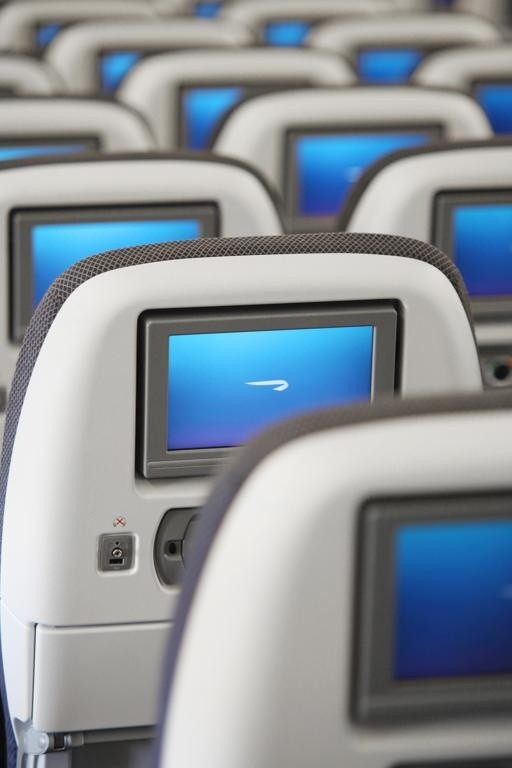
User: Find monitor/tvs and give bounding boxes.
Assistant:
[144,309,399,477]
[259,17,311,45]
[35,22,62,50]
[13,206,217,344]
[188,2,221,16]
[353,44,426,84]
[285,124,443,234]
[437,192,512,314]
[96,46,145,93]
[348,494,512,723]
[0,138,100,165]
[472,80,512,134]
[178,84,253,152]
